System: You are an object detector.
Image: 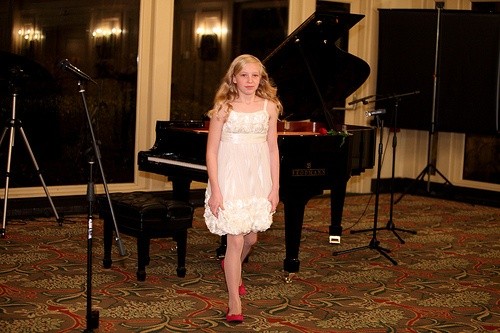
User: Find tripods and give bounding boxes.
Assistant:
[0,87,63,237]
[394,7,456,203]
[349,89,419,245]
[331,116,399,266]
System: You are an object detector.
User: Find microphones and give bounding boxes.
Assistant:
[348,95,374,105]
[365,109,385,116]
[60,59,98,85]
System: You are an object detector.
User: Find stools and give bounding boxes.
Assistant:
[97,192,194,282]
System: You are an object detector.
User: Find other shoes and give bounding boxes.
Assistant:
[221,258,246,295]
[225,304,244,322]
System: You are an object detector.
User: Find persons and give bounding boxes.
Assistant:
[203,54,283,324]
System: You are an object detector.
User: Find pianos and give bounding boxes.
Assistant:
[137,10,377,284]
